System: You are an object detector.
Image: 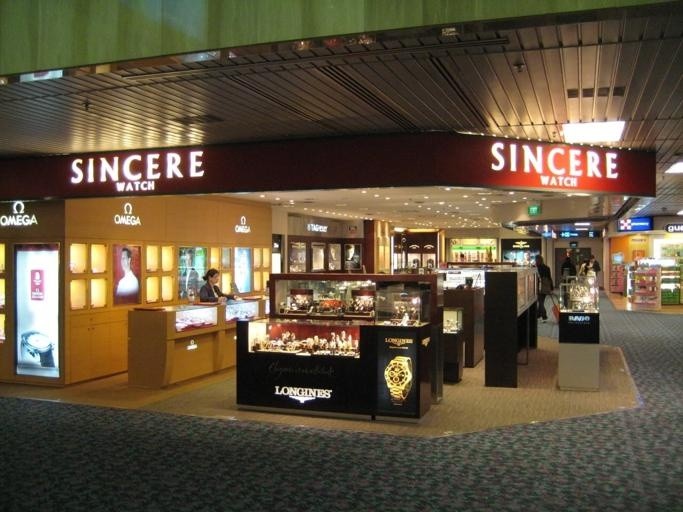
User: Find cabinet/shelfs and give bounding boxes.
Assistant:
[127,239,600,420]
[607,239,682,311]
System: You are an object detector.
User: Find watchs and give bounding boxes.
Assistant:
[383,356,413,405]
[20,332,55,367]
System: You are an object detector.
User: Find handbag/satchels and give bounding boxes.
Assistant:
[537,270,552,295]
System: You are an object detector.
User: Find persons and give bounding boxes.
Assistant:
[456,277,473,289]
[199,269,238,302]
[578,259,587,275]
[533,255,555,323]
[584,256,600,275]
[330,245,339,261]
[179,250,198,298]
[116,248,138,294]
[560,257,577,306]
[345,244,354,261]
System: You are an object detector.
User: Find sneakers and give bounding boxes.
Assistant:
[538,316,548,323]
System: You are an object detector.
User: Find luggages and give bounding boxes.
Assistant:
[550,294,559,320]
